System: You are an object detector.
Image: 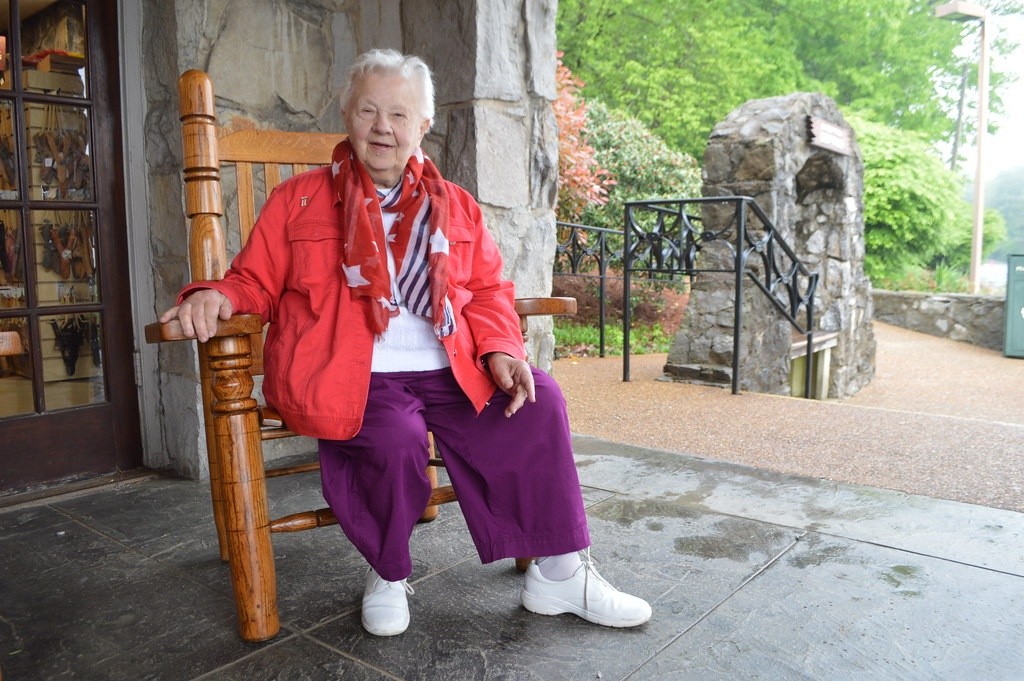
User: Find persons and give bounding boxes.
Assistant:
[161,49,653,636]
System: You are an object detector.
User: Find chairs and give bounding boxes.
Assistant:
[145,67,577,645]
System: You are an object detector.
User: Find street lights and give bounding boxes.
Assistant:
[935,1,994,293]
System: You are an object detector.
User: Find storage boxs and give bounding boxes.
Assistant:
[3,53,84,91]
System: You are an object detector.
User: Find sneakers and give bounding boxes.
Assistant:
[521,545,652,627]
[362,566,414,635]
